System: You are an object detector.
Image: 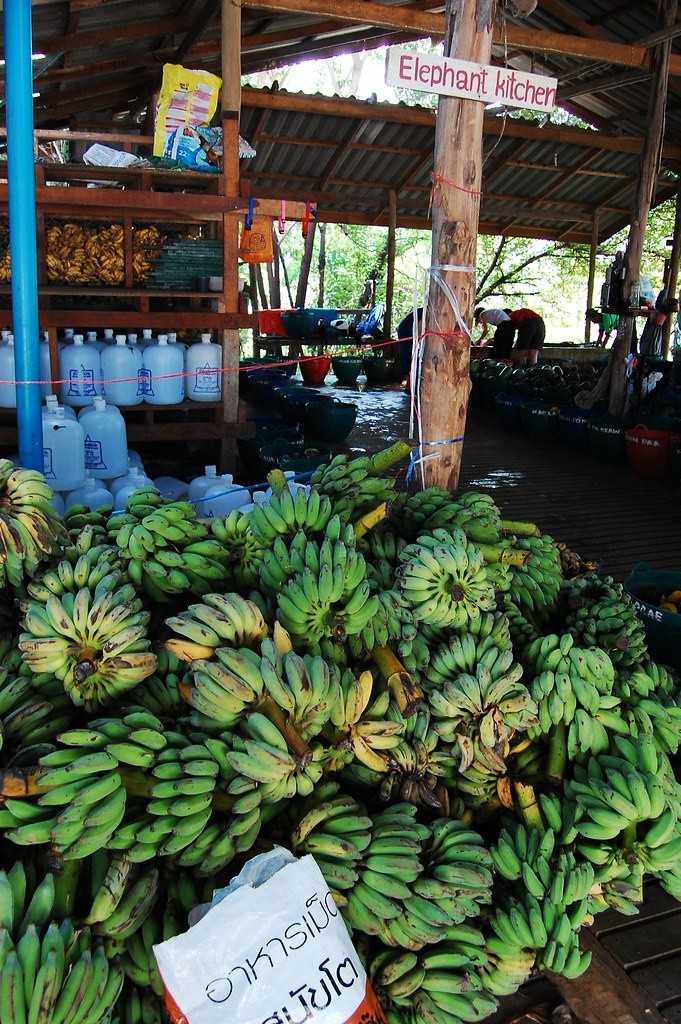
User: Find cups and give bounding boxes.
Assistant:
[655,312,666,326]
[665,298,679,312]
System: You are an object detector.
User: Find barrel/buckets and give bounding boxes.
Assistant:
[273,384,358,442]
[298,356,395,384]
[470,377,681,478]
[241,426,304,469]
[560,546,602,580]
[245,413,299,434]
[281,314,313,336]
[494,358,513,367]
[239,355,297,377]
[538,358,574,365]
[623,561,681,665]
[259,438,333,478]
[248,375,290,406]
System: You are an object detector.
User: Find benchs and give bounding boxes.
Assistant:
[255,308,387,375]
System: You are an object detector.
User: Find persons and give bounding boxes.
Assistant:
[396,305,423,395]
[503,308,545,366]
[588,308,637,354]
[473,306,516,362]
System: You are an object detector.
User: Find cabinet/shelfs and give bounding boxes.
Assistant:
[0,160,261,483]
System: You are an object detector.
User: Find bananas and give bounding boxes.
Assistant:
[0,223,198,287]
[0,440,681,1024]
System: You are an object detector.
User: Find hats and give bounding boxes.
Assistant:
[473,308,485,327]
[586,308,598,319]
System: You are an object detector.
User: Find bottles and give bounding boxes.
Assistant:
[0,330,18,408]
[630,280,641,306]
[153,477,190,501]
[190,466,307,516]
[42,395,154,519]
[41,329,221,405]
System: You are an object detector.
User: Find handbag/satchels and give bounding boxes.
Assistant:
[240,216,274,263]
[640,318,663,355]
